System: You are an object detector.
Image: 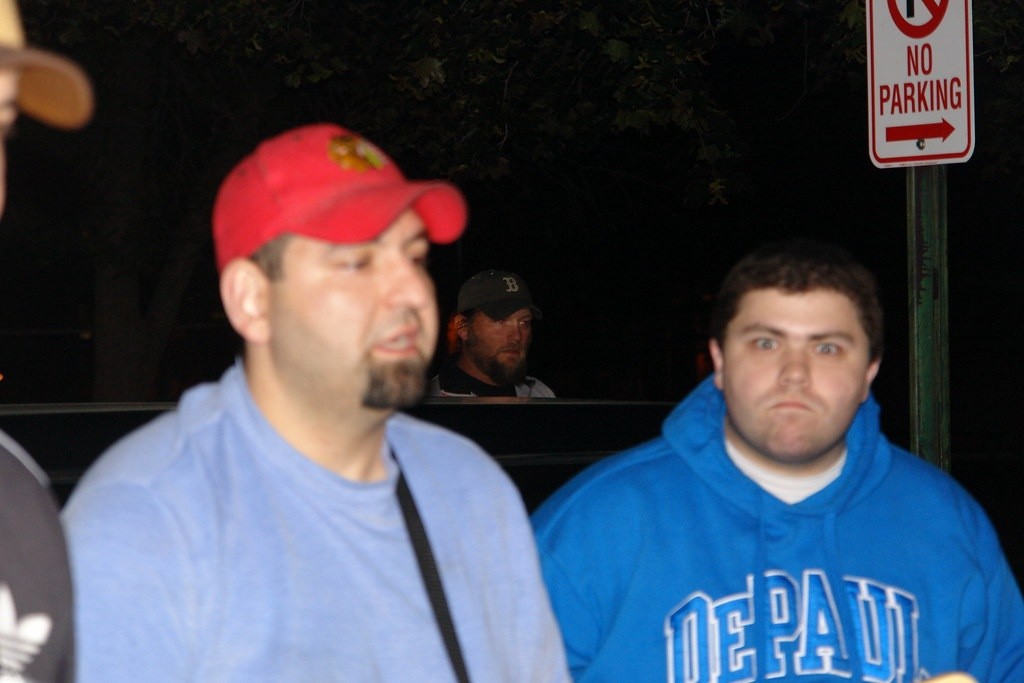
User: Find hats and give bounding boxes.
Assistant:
[210,121,468,274]
[1,0,95,129]
[458,269,543,321]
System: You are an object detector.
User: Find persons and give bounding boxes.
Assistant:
[530,249,1024,683]
[427,269,557,398]
[0,0,91,683]
[59,123,575,683]
